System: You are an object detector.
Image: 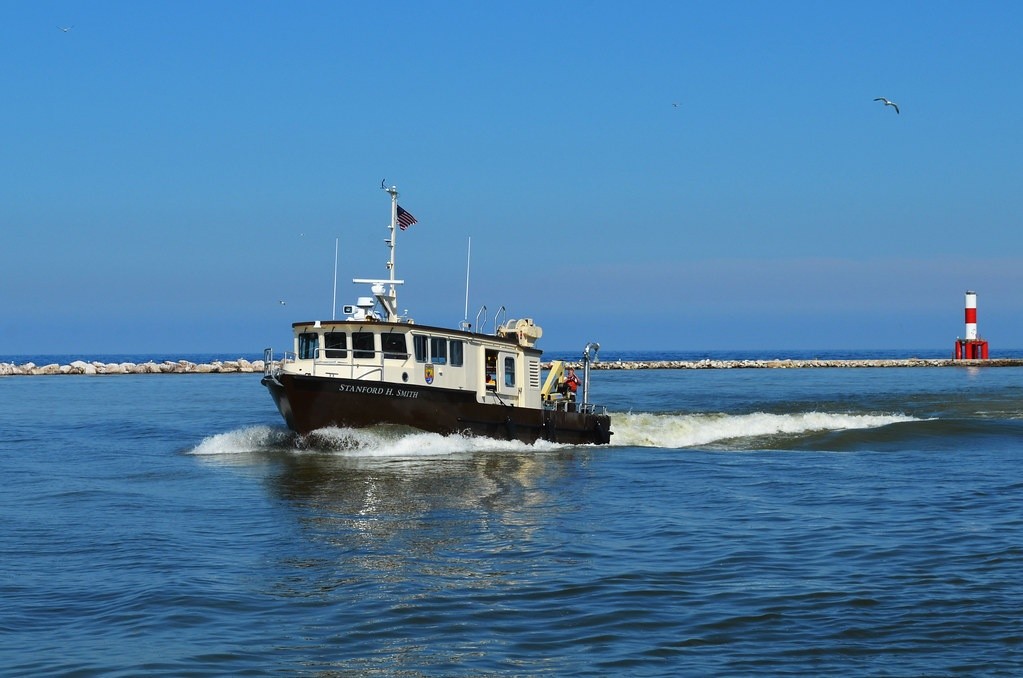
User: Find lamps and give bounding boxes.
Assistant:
[312,320,326,329]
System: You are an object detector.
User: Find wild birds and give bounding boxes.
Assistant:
[873,97,900,115]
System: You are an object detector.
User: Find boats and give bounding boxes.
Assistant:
[259,174,617,447]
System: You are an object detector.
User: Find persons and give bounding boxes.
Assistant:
[564,368,582,411]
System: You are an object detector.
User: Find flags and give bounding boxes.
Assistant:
[396,204,417,232]
